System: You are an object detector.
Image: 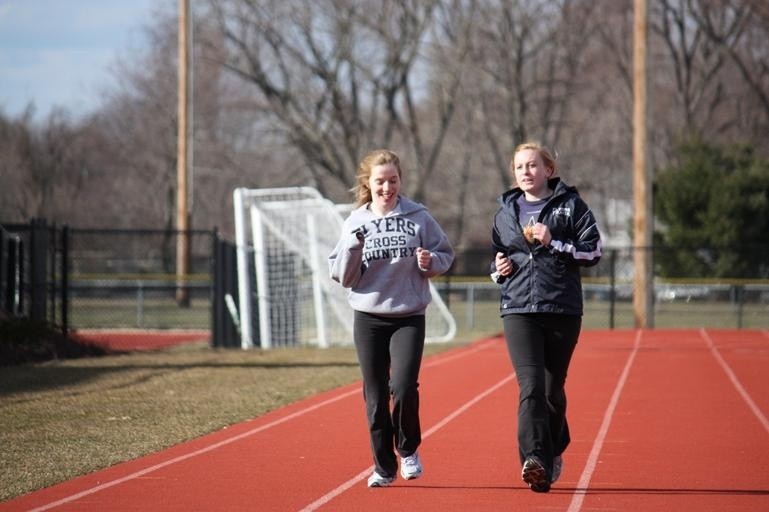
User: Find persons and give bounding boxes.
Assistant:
[489,142,606,493]
[329,146,455,489]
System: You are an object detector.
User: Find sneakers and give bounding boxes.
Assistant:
[521,457,550,492]
[550,455,563,483]
[400,450,424,481]
[367,471,397,487]
